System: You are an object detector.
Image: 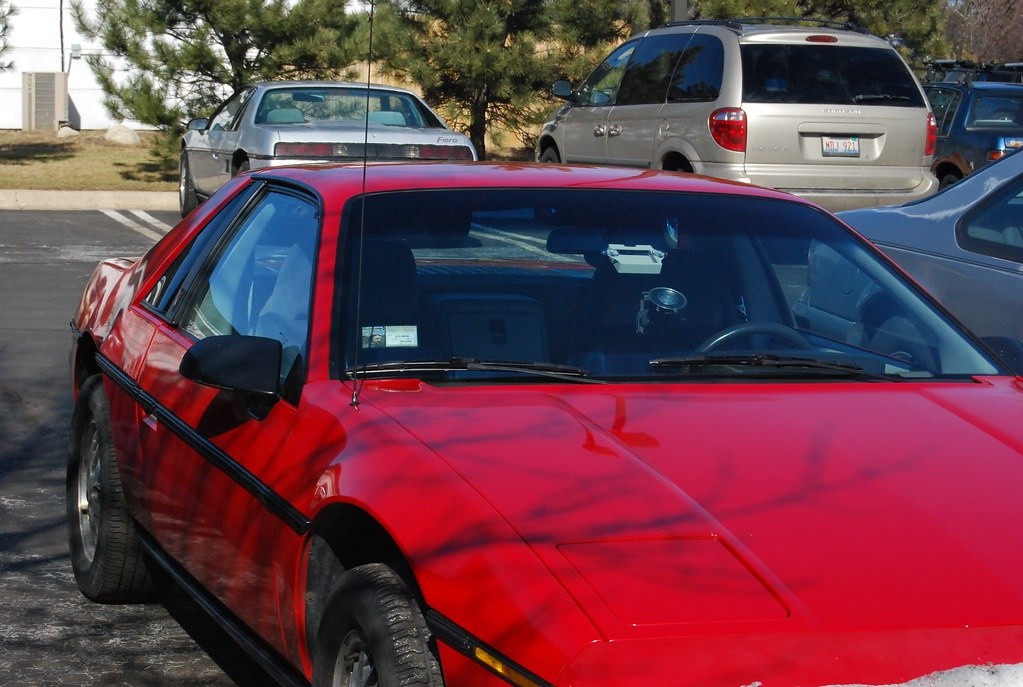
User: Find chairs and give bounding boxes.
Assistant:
[365,112,407,126]
[335,238,448,362]
[569,246,733,361]
[266,108,305,124]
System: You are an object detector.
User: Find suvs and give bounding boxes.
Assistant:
[911,57,1023,198]
[531,13,940,263]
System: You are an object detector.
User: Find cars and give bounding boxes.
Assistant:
[794,142,1022,380]
[63,159,1022,687]
[177,83,480,247]
